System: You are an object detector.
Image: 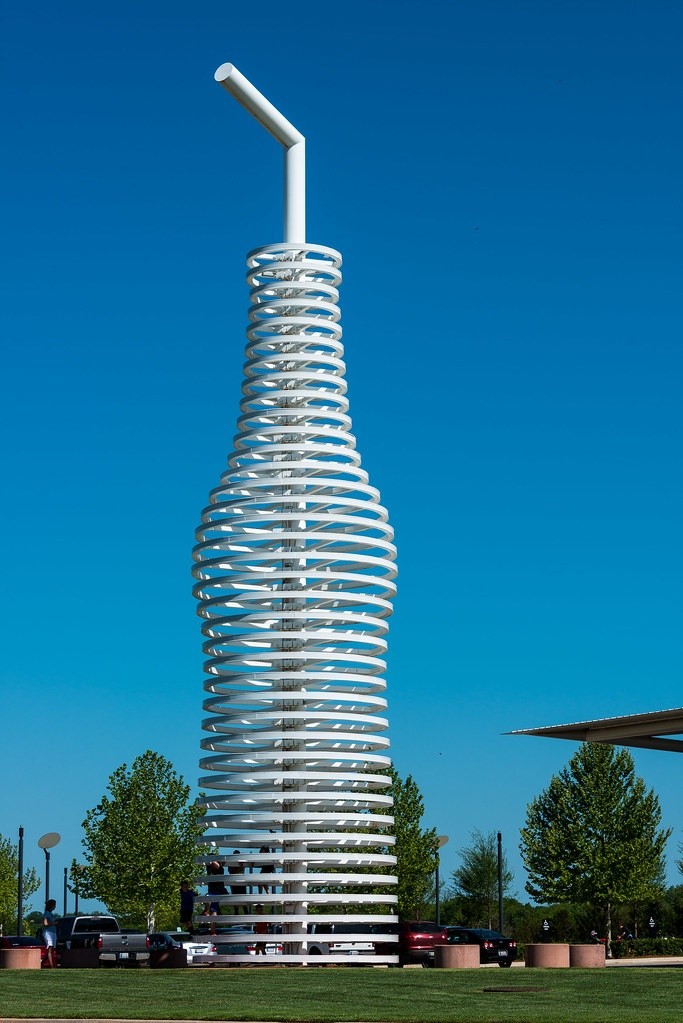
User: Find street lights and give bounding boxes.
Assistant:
[38,832,61,902]
[431,835,449,926]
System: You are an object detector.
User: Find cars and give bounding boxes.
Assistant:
[445,926,519,968]
[189,927,284,969]
[146,930,218,969]
[0,936,58,969]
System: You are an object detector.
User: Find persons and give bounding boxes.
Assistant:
[258,845,275,894]
[42,899,61,967]
[591,931,602,945]
[255,904,271,955]
[618,924,632,939]
[179,882,198,932]
[205,853,228,935]
[228,850,249,915]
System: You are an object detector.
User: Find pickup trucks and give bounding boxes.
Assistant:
[36,915,152,970]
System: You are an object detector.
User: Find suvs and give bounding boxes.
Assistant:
[367,919,449,970]
[263,922,376,968]
